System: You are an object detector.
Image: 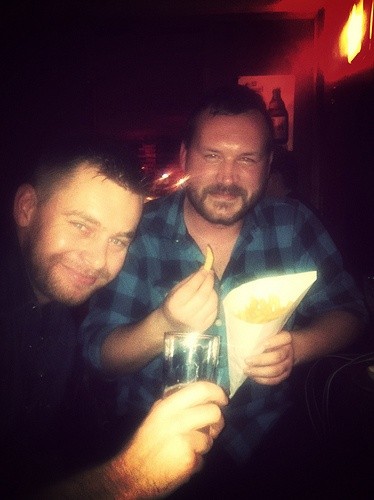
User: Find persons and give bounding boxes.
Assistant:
[74,85,373,500]
[1,119,228,500]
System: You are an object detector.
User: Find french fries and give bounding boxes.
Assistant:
[232,298,289,323]
[202,245,215,274]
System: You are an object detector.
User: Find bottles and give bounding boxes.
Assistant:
[267,87,288,147]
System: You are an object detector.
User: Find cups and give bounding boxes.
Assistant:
[161,330,222,436]
